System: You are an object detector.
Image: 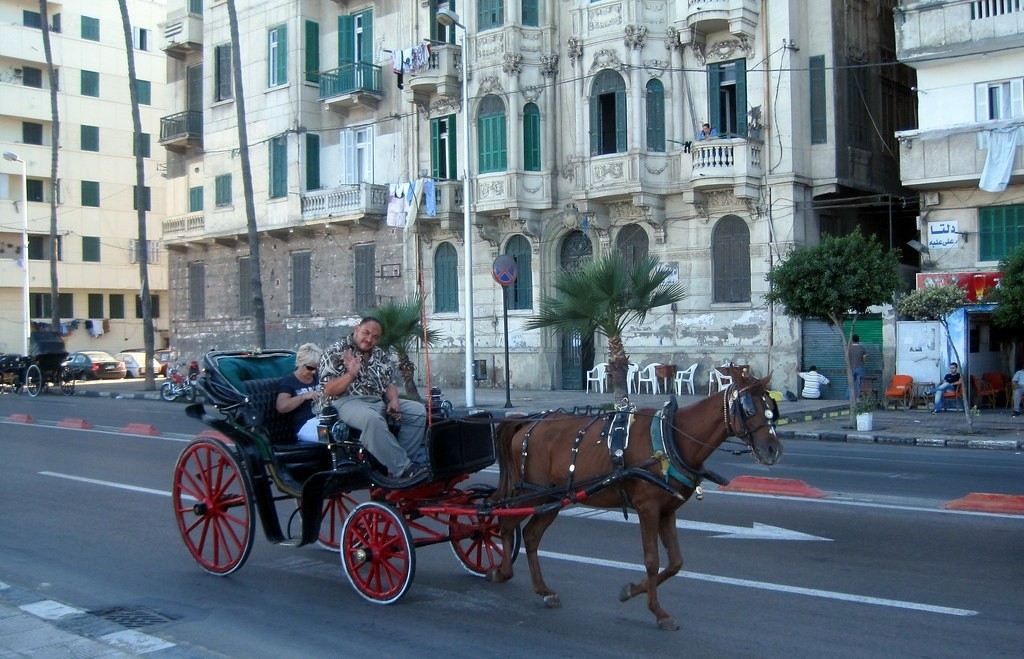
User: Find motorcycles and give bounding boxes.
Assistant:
[160,361,199,402]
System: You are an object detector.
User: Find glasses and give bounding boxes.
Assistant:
[304,364,318,371]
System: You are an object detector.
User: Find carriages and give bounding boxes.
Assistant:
[171,346,787,630]
[0,331,75,397]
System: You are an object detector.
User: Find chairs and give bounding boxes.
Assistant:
[625,363,662,395]
[936,377,963,413]
[713,368,732,392]
[884,375,913,411]
[671,362,698,395]
[585,363,608,394]
[970,371,1011,409]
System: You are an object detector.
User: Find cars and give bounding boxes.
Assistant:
[66,351,126,380]
[112,352,162,378]
[154,349,169,377]
[0,354,25,384]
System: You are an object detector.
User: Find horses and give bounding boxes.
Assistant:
[486,364,783,632]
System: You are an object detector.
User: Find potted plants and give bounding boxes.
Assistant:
[853,391,878,433]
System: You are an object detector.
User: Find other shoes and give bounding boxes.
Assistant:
[923,392,932,397]
[1011,411,1019,416]
[932,410,940,414]
[856,398,862,402]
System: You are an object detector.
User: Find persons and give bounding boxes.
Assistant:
[187,361,200,380]
[922,363,963,415]
[696,124,719,141]
[798,366,833,400]
[1011,362,1024,417]
[319,317,431,486]
[844,334,868,402]
[277,343,356,468]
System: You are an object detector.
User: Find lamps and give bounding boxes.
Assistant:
[908,240,930,264]
[910,87,928,95]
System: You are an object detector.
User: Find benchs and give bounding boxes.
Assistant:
[242,373,329,485]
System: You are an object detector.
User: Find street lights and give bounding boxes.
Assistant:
[5,152,31,359]
[435,7,475,407]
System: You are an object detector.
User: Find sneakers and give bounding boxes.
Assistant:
[400,464,427,479]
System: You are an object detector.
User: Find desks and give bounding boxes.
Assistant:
[912,381,935,414]
